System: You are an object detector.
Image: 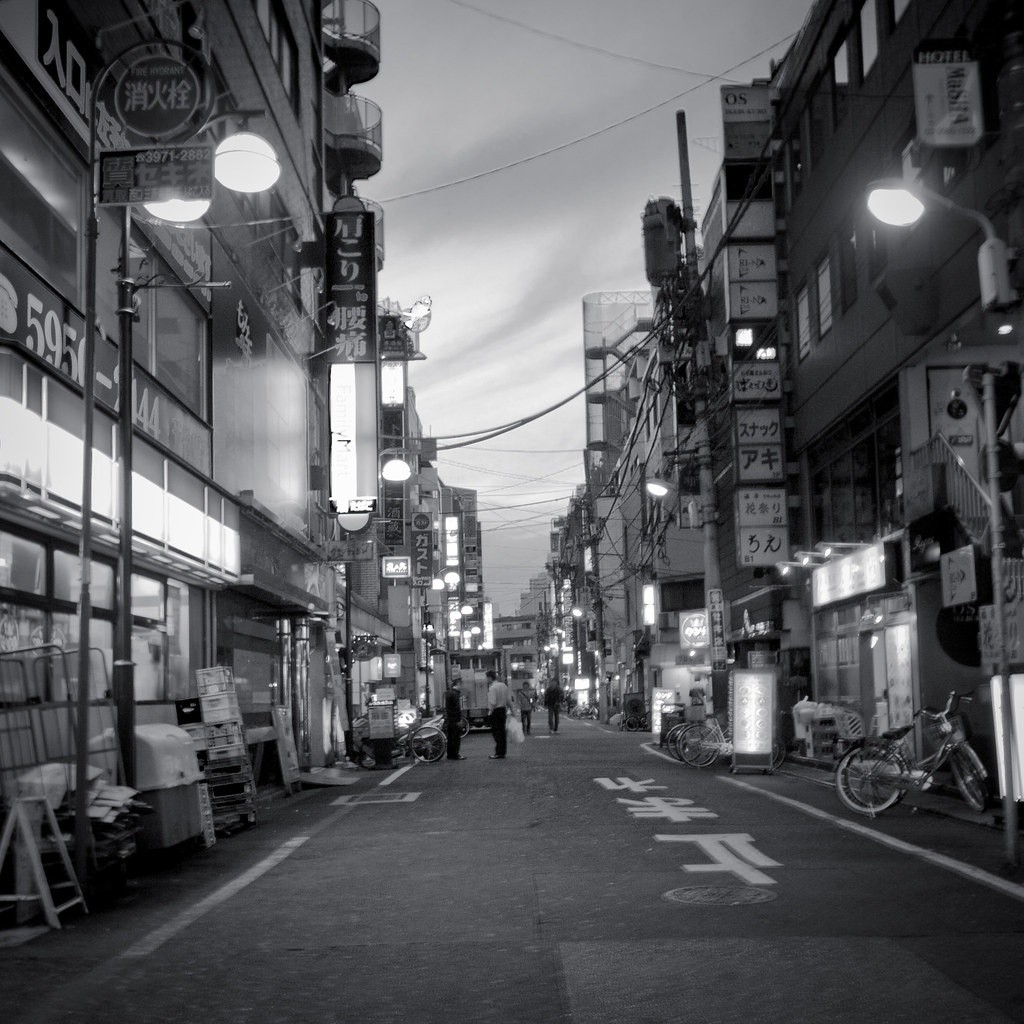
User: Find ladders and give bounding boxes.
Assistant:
[0,794,89,930]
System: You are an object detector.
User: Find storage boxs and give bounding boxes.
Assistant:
[811,717,839,760]
[179,666,257,815]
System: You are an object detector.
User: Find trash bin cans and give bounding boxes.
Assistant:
[85,724,205,851]
[568,699,577,715]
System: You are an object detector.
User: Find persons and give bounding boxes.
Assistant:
[483,670,516,759]
[517,681,538,736]
[543,678,563,735]
[444,678,467,760]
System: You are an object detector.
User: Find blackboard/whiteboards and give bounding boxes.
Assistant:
[660,703,686,736]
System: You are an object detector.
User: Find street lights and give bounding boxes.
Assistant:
[424,564,461,719]
[70,100,290,912]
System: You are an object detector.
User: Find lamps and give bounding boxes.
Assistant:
[775,561,822,575]
[794,551,844,565]
[815,541,868,557]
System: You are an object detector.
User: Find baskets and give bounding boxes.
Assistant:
[685,704,706,722]
[925,716,967,751]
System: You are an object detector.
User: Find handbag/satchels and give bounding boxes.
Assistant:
[506,716,525,746]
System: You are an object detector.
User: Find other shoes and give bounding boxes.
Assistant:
[549,730,553,734]
[555,730,560,733]
[489,755,506,759]
[459,756,467,759]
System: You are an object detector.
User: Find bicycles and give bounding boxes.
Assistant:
[572,702,601,721]
[625,708,652,733]
[667,708,787,772]
[357,718,448,769]
[832,688,994,819]
